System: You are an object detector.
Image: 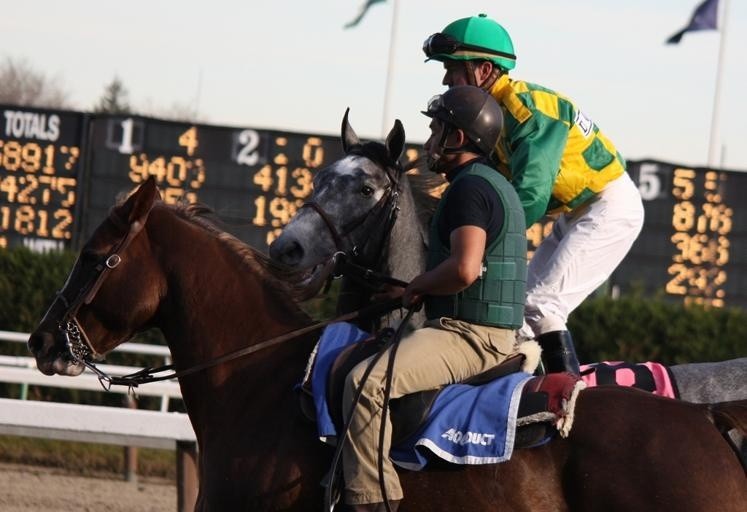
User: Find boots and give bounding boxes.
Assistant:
[535,330,581,374]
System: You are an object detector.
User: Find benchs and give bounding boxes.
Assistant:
[0,330,200,512]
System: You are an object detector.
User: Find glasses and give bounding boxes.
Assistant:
[425,94,468,128]
[423,32,514,58]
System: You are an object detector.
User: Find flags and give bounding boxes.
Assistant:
[663,1,719,44]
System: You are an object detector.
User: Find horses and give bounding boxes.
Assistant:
[268,106,747,404]
[26,174,747,512]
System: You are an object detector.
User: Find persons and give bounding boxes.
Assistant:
[337,87,528,509]
[424,13,645,376]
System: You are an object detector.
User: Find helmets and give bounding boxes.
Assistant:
[424,13,515,74]
[419,84,504,157]
